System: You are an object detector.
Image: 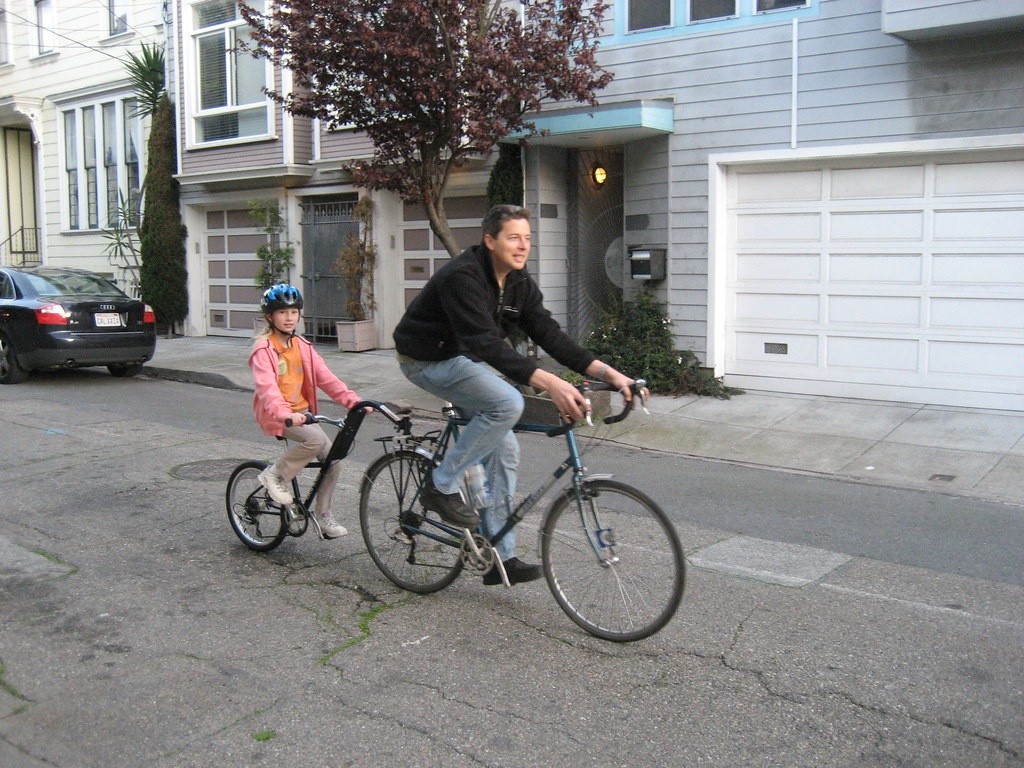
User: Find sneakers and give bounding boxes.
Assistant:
[312,515,349,538]
[257,464,293,505]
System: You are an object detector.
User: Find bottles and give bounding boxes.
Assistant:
[464,460,493,508]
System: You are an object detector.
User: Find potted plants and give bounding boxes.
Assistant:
[247,200,297,335]
[330,197,379,351]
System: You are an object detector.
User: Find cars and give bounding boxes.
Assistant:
[0,264,157,385]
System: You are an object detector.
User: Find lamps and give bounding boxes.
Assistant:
[590,161,608,191]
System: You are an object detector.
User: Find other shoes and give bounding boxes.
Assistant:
[419,479,479,529]
[482,558,546,587]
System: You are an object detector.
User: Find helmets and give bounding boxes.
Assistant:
[260,283,304,314]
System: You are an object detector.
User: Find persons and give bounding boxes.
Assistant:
[248,283,373,538]
[393,205,653,584]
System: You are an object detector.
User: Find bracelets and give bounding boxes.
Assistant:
[600,364,608,382]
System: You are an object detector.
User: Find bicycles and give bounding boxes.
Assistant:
[225,379,688,643]
